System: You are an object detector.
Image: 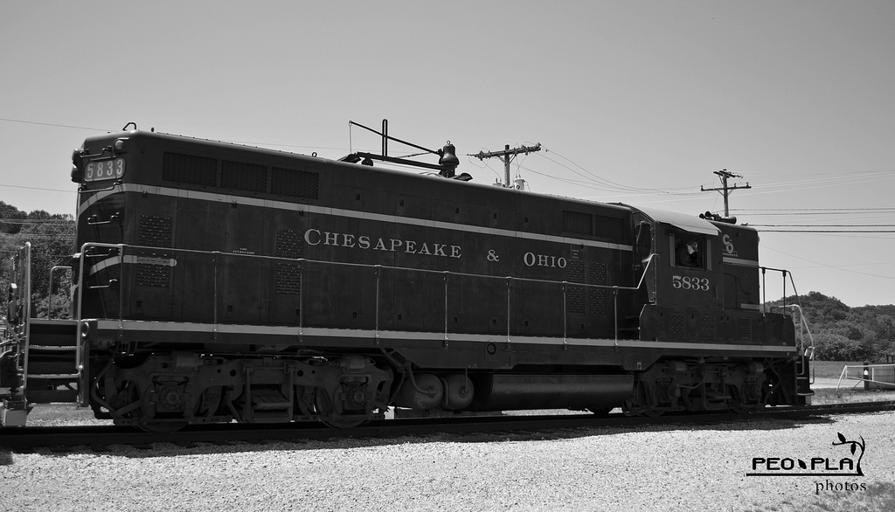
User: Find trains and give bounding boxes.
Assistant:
[68,129,817,431]
